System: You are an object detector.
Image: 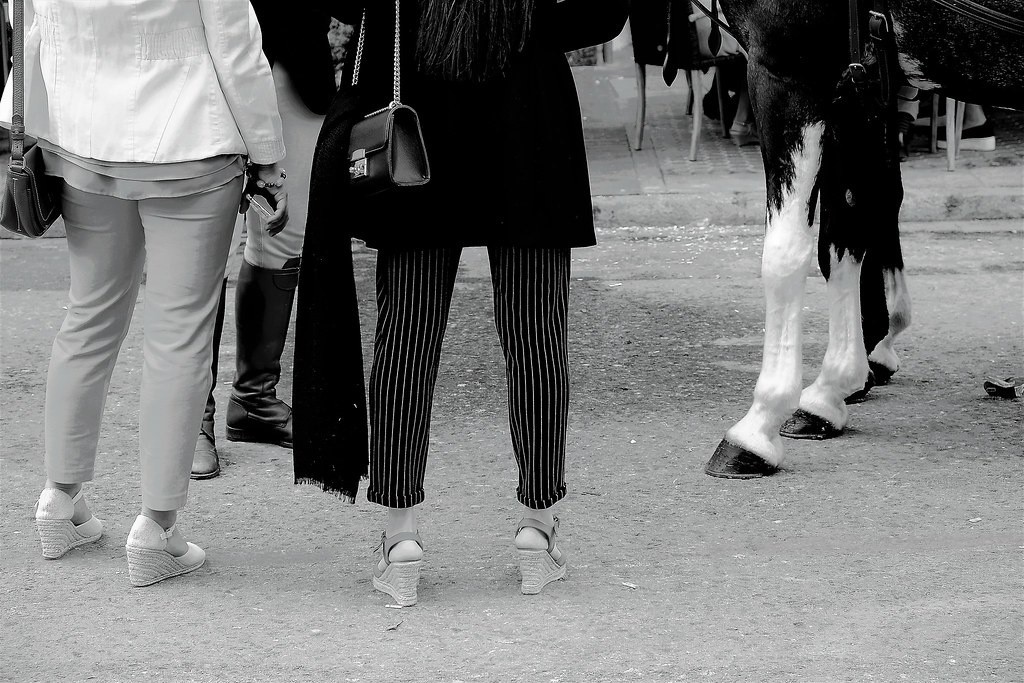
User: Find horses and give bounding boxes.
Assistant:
[700,0,1024,481]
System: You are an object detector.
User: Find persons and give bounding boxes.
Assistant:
[691,0,996,162]
[310,0,634,603]
[1,0,283,583]
[185,0,299,478]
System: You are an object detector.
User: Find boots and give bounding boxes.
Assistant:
[189,276,228,479]
[226,255,302,448]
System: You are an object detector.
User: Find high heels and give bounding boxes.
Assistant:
[373,529,423,607]
[515,516,566,595]
[126,515,206,587]
[34,488,103,560]
[729,121,760,146]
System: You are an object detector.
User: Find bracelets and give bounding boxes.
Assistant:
[243,162,287,189]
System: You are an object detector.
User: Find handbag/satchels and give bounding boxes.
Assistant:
[347,105,430,186]
[0,142,62,238]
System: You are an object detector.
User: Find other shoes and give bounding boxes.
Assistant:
[936,119,996,151]
[896,118,914,161]
[910,105,966,126]
[703,89,740,122]
